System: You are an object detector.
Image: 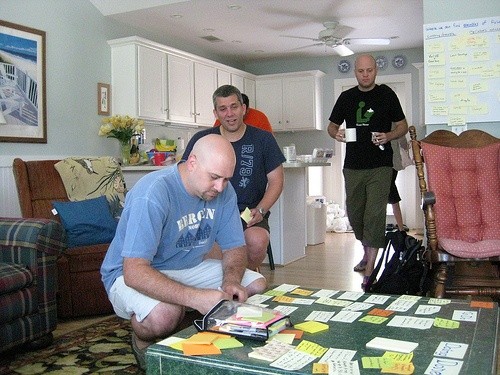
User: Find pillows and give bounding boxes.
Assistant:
[51,196,118,247]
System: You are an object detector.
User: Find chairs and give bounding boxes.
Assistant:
[407,125,500,301]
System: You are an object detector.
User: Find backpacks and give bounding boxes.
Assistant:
[366,230,424,295]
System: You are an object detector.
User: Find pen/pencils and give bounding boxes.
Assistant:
[217,286,227,294]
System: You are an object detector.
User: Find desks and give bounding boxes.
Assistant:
[145,285,497,375]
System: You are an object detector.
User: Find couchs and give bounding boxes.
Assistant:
[0,217,63,353]
[13,158,115,319]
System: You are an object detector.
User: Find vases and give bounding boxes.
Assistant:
[119,139,134,166]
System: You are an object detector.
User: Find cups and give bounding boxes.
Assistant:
[154,153,165,165]
[313,148,334,163]
[340,128,356,142]
[283,144,296,163]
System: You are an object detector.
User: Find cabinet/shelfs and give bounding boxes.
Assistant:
[107,36,325,132]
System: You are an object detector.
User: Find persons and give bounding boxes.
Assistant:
[214,94,273,134]
[182,84,287,271]
[100,134,267,371]
[388,168,406,232]
[327,54,409,287]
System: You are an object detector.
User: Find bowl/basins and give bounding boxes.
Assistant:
[147,152,175,159]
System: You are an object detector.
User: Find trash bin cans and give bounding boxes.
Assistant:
[306,203,324,245]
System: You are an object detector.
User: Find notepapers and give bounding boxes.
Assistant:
[182,343,222,356]
[214,337,244,350]
[242,312,275,323]
[293,321,330,334]
[190,333,218,345]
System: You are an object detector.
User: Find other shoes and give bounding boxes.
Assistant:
[354,260,367,271]
[132,332,157,371]
[361,276,377,287]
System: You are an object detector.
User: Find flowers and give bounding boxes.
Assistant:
[98,114,145,163]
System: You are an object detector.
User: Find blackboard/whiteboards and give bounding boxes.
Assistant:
[425,17,500,125]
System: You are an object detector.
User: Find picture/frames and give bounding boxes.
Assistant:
[0,20,48,143]
[97,83,110,115]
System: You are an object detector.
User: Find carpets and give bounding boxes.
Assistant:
[0,311,201,375]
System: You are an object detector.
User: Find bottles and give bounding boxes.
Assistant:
[176,137,185,161]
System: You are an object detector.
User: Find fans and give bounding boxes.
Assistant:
[280,21,389,57]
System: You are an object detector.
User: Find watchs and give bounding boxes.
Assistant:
[256,206,267,216]
